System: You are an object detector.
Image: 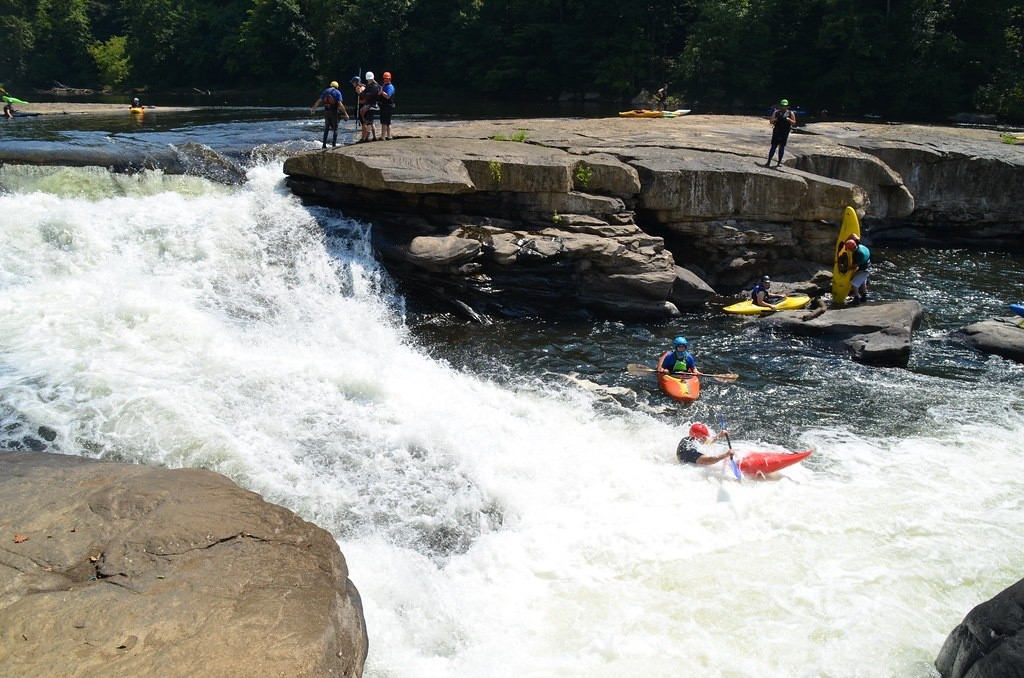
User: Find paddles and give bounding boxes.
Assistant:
[625,362,738,382]
[719,412,742,481]
[118,106,156,108]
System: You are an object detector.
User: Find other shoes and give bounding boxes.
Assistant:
[356,135,393,143]
[860,294,867,302]
[848,297,859,305]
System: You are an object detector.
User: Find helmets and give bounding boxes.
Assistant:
[383,72,391,80]
[689,425,709,438]
[846,240,856,251]
[762,275,770,283]
[673,337,688,355]
[805,285,817,298]
[8,100,13,104]
[349,76,361,83]
[134,97,139,103]
[330,81,340,88]
[780,99,789,106]
[365,72,374,80]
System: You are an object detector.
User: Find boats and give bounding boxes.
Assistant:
[722,292,811,320]
[130,107,143,115]
[618,108,682,118]
[829,205,862,304]
[701,450,814,480]
[1,95,29,104]
[662,109,692,116]
[655,350,701,405]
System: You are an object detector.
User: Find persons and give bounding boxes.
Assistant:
[660,337,703,376]
[843,233,872,307]
[4,100,20,117]
[765,100,795,167]
[311,81,349,148]
[355,72,380,144]
[657,84,668,111]
[350,76,370,142]
[677,424,735,466]
[378,72,395,141]
[751,276,786,309]
[132,98,141,107]
